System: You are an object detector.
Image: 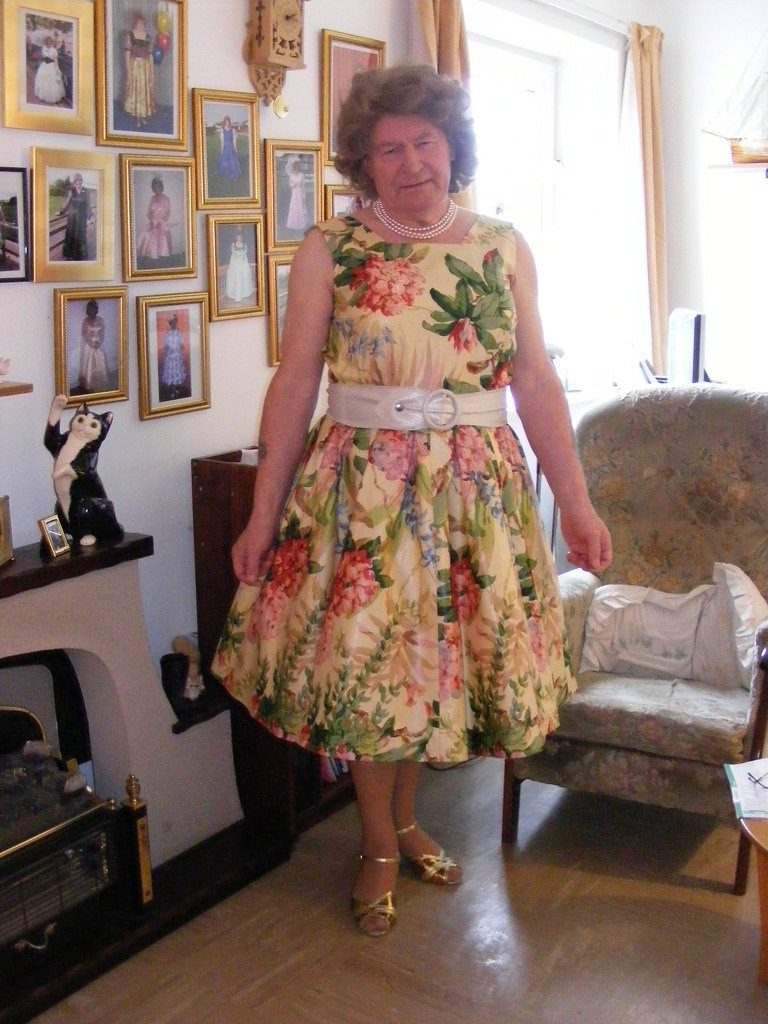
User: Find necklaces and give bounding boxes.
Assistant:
[373,198,457,240]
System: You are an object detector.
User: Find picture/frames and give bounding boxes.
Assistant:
[0,167,31,283]
[320,28,386,167]
[264,140,325,253]
[192,88,262,210]
[119,153,198,283]
[205,213,266,321]
[54,285,130,410]
[135,291,211,421]
[30,145,116,284]
[94,0,188,152]
[37,514,70,558]
[266,255,294,367]
[325,185,362,221]
[0,0,93,136]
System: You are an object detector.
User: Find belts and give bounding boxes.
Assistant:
[326,376,508,432]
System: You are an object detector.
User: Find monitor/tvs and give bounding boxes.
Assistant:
[667,308,712,388]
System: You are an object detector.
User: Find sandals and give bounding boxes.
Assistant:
[396,821,463,885]
[350,854,400,937]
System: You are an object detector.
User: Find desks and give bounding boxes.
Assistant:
[738,789,768,990]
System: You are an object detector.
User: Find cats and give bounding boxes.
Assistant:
[44,394,124,544]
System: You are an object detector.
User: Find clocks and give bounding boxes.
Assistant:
[242,0,311,118]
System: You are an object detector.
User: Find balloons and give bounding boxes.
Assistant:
[154,11,170,34]
[152,46,164,65]
[157,32,169,53]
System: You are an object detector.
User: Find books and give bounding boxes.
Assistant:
[320,754,349,783]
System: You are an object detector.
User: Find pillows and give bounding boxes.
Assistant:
[579,561,768,690]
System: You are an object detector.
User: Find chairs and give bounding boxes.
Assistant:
[500,383,768,897]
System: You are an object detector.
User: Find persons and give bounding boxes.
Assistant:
[137,178,173,259]
[224,227,256,302]
[209,62,613,937]
[121,14,157,128]
[287,162,309,230]
[55,174,96,260]
[34,37,67,103]
[161,314,187,396]
[217,116,241,180]
[78,299,109,392]
